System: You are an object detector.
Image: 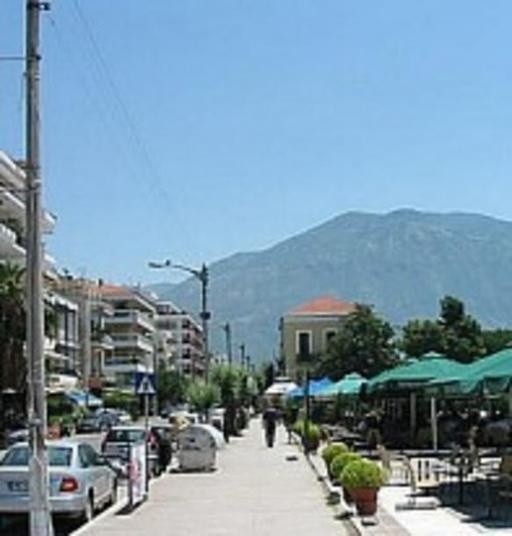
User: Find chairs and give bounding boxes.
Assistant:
[375,441,512,524]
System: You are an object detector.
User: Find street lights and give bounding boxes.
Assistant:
[146,258,214,425]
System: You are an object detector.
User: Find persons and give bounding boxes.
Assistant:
[262,402,278,446]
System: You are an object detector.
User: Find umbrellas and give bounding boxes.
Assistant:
[287,341,512,456]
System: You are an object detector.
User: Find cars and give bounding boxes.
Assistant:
[149,397,248,451]
[0,438,119,524]
[3,406,133,446]
[101,424,173,480]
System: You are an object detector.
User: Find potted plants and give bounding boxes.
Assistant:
[321,440,388,516]
